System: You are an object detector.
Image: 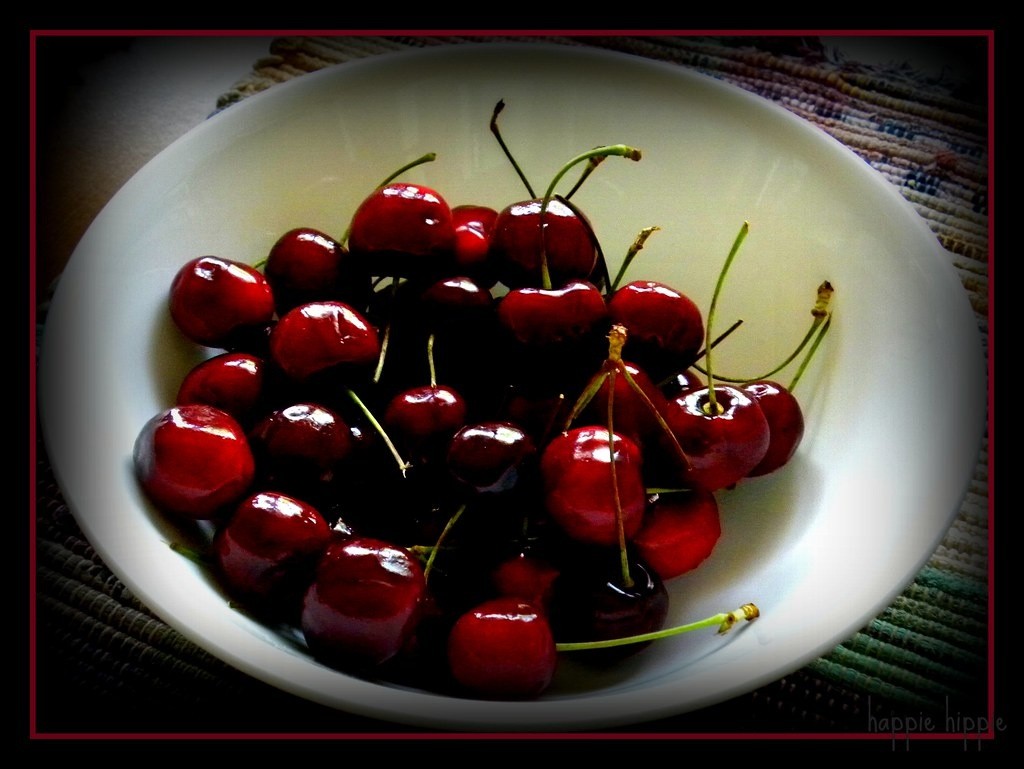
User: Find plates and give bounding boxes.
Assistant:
[39,43,987,728]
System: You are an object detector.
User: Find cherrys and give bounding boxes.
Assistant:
[135,96,841,686]
